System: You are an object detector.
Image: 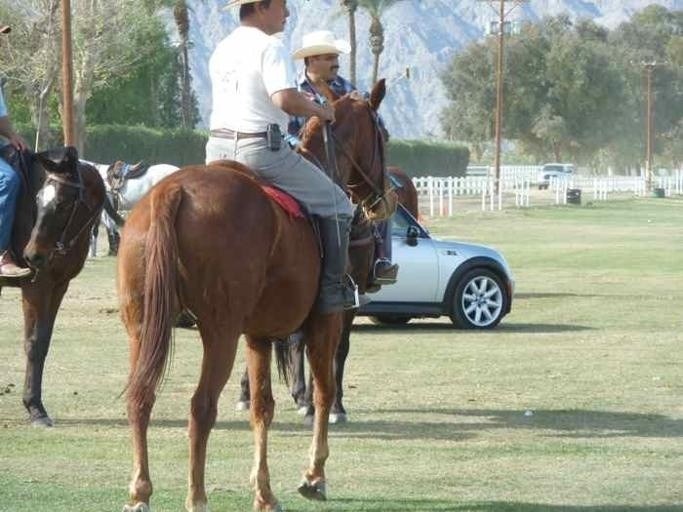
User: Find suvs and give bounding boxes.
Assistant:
[536,164,572,189]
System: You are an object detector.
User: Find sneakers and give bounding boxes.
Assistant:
[0,250,30,277]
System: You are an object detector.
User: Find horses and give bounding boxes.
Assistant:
[77,158,180,257]
[0,146,105,428]
[103,78,418,512]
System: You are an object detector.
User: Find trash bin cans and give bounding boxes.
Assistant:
[567,189,581,204]
[654,188,665,197]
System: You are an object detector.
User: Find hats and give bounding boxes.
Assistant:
[0,22,11,36]
[222,1,259,12]
[289,30,352,60]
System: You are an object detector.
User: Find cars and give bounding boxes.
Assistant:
[354,203,514,329]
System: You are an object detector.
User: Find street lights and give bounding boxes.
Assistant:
[632,61,665,185]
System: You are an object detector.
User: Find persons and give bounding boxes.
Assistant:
[0,24,33,277]
[282,29,391,151]
[203,1,371,315]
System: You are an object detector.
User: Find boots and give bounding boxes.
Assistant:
[318,218,364,305]
